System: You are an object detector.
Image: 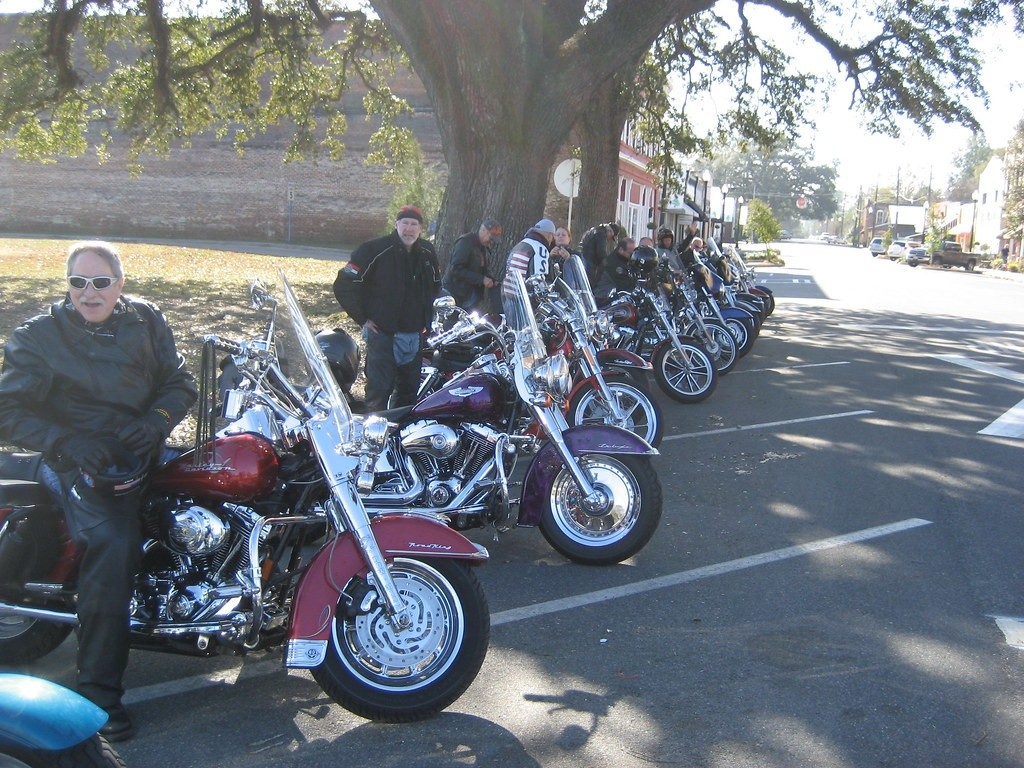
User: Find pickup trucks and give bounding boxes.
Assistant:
[907,241,981,271]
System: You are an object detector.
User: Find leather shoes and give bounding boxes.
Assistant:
[97,707,133,743]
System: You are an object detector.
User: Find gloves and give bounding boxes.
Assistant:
[56,435,113,475]
[114,411,171,456]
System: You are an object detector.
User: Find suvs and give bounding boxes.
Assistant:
[887,240,921,261]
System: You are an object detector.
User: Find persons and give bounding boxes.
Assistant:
[0,245,198,743]
[442,218,701,331]
[333,205,442,413]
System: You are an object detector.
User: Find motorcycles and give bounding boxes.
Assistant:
[203,243,664,567]
[530,237,776,450]
[0,672,127,768]
[0,260,490,724]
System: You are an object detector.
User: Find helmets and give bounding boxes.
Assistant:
[657,227,673,246]
[315,328,362,394]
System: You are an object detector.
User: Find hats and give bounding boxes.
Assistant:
[483,218,502,244]
[397,206,422,223]
[608,223,619,241]
[534,219,556,236]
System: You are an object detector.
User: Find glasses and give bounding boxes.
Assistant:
[67,275,118,291]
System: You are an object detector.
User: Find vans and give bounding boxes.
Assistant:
[869,238,886,258]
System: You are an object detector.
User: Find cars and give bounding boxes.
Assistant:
[778,230,791,239]
[821,232,845,244]
